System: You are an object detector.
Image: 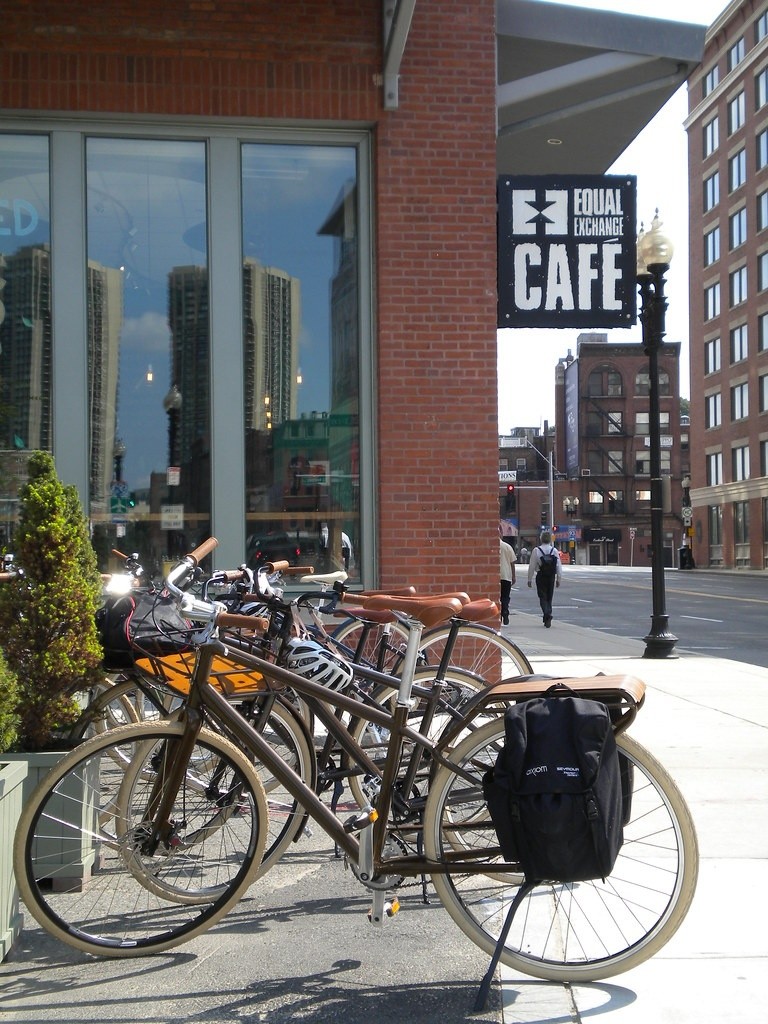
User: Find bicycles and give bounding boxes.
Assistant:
[13,536,699,1013]
[64,549,534,904]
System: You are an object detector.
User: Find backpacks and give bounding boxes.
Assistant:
[537,547,557,578]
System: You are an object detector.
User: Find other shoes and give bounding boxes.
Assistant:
[544,615,553,628]
[503,611,509,625]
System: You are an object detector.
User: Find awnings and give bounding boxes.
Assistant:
[496,0,707,174]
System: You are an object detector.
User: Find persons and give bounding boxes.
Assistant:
[527,530,563,628]
[521,546,527,564]
[500,538,518,625]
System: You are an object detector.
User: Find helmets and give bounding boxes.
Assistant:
[287,637,355,692]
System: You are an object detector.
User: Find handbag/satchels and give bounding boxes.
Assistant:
[482,682,623,881]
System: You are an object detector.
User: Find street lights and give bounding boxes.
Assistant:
[564,497,579,564]
[113,440,126,571]
[638,208,681,659]
[681,475,692,559]
[163,384,183,560]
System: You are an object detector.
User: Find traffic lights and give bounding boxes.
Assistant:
[553,526,557,532]
[507,484,515,497]
